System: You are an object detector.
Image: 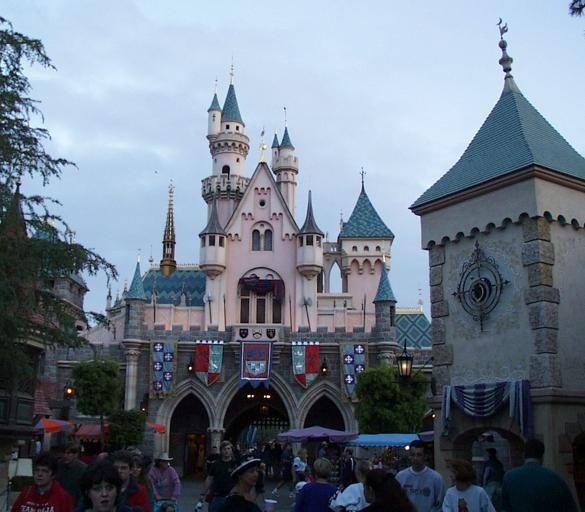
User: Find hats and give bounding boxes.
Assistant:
[154,452,173,461]
[227,447,262,478]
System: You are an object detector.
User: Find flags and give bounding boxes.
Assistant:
[144,340,368,402]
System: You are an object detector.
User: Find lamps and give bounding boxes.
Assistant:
[187,357,193,373]
[320,356,329,377]
[64,378,75,400]
[391,337,436,396]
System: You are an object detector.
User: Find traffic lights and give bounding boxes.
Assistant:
[64,385,78,400]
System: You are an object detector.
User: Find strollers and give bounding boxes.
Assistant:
[152,497,181,512]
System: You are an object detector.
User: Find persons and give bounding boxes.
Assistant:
[194,428,577,511]
[6,433,181,512]
